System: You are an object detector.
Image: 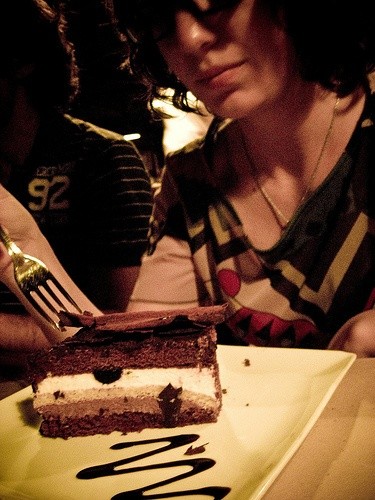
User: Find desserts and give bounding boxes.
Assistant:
[28,302,227,440]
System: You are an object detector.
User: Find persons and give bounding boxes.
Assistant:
[0,0,375,357]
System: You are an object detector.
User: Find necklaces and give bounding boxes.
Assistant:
[236,96,340,223]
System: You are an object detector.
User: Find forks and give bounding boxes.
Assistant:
[0,227,82,333]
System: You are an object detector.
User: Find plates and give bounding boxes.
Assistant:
[0,341,357,500]
